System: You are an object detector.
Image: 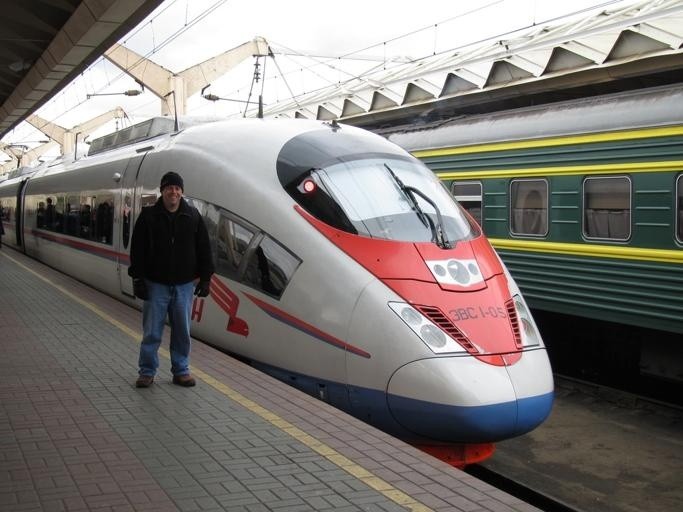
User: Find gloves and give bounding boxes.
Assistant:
[130,276,150,300]
[193,274,211,299]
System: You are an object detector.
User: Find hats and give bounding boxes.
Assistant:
[159,171,185,194]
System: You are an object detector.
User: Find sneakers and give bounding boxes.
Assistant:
[172,373,196,387]
[135,374,154,389]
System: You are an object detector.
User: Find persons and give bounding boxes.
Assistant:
[126,171,214,389]
[36,197,128,249]
